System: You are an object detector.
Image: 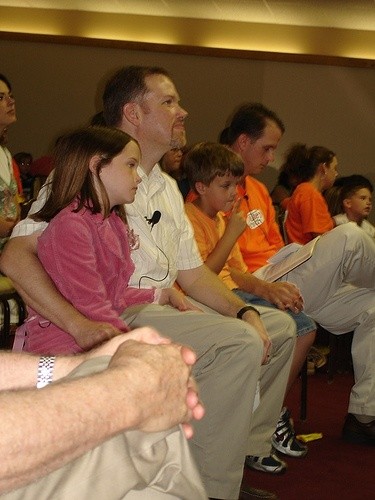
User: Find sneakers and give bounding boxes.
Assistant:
[341,413,375,445]
[270,407,308,456]
[245,452,287,474]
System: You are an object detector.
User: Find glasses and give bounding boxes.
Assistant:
[0,95,16,103]
[20,162,30,166]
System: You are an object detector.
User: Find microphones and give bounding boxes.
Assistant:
[148,211,161,223]
[244,193,248,200]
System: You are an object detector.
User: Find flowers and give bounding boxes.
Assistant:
[126,229,140,250]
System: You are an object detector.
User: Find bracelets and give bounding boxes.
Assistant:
[237,306,260,319]
[36,356,56,388]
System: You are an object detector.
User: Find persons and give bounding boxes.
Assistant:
[271,146,338,246]
[13,126,204,356]
[184,142,316,474]
[0,75,28,328]
[332,175,375,237]
[0,65,297,500]
[14,152,32,202]
[0,326,205,500]
[185,104,375,446]
